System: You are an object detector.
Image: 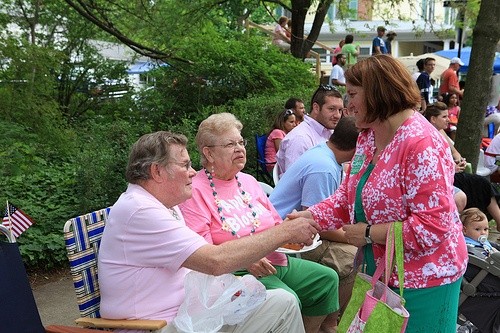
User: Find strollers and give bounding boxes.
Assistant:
[456,240,500,333]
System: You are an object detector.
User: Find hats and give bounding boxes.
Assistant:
[378,26,387,31]
[451,57,464,66]
[387,31,397,36]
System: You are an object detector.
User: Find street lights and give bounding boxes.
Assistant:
[443,0,468,81]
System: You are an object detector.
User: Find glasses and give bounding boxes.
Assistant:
[284,109,294,117]
[208,139,247,149]
[166,161,192,171]
[312,83,338,102]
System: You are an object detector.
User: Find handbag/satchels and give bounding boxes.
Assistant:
[336,222,410,333]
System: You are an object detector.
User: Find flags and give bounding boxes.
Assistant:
[2,201,34,239]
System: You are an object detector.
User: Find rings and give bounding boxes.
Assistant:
[253,273,256,276]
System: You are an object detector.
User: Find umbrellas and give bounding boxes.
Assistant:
[435,47,500,72]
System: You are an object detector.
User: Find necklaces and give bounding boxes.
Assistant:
[204,167,256,238]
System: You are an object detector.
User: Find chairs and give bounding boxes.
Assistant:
[0,233,115,333]
[66,205,168,333]
[253,133,277,188]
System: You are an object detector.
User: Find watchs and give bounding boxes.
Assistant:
[365,225,374,244]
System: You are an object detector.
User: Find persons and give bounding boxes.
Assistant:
[288,53,467,333]
[177,111,339,333]
[263,17,500,312]
[96,131,319,333]
